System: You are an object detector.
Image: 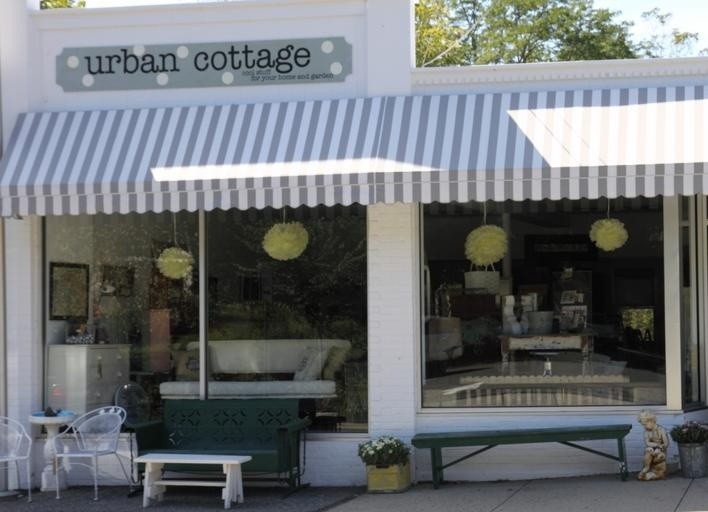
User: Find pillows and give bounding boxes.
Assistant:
[321,346,350,382]
[291,347,327,383]
[169,346,215,382]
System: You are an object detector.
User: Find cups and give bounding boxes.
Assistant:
[527,311,555,336]
[559,310,585,334]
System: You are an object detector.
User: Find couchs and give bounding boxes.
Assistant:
[158,338,352,401]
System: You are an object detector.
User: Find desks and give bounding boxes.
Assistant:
[26,415,77,493]
[495,332,594,363]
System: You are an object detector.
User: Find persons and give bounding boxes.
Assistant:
[635,410,671,482]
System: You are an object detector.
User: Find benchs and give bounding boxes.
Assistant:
[122,397,314,502]
[131,452,253,511]
[409,423,633,490]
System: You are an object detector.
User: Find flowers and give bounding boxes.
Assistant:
[261,221,309,262]
[155,247,194,280]
[667,419,707,444]
[587,217,628,253]
[462,224,509,267]
[356,436,412,470]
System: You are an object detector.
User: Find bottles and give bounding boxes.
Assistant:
[48,383,63,412]
[434,283,452,318]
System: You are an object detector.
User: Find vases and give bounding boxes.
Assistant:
[362,460,410,493]
[674,440,708,479]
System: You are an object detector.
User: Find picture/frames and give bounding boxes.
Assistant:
[559,290,576,305]
[115,381,151,427]
[101,263,135,298]
[47,260,90,322]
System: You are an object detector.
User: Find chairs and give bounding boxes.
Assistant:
[0,417,33,504]
[51,405,133,502]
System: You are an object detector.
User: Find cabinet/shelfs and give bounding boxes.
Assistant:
[48,343,132,417]
[126,309,171,374]
